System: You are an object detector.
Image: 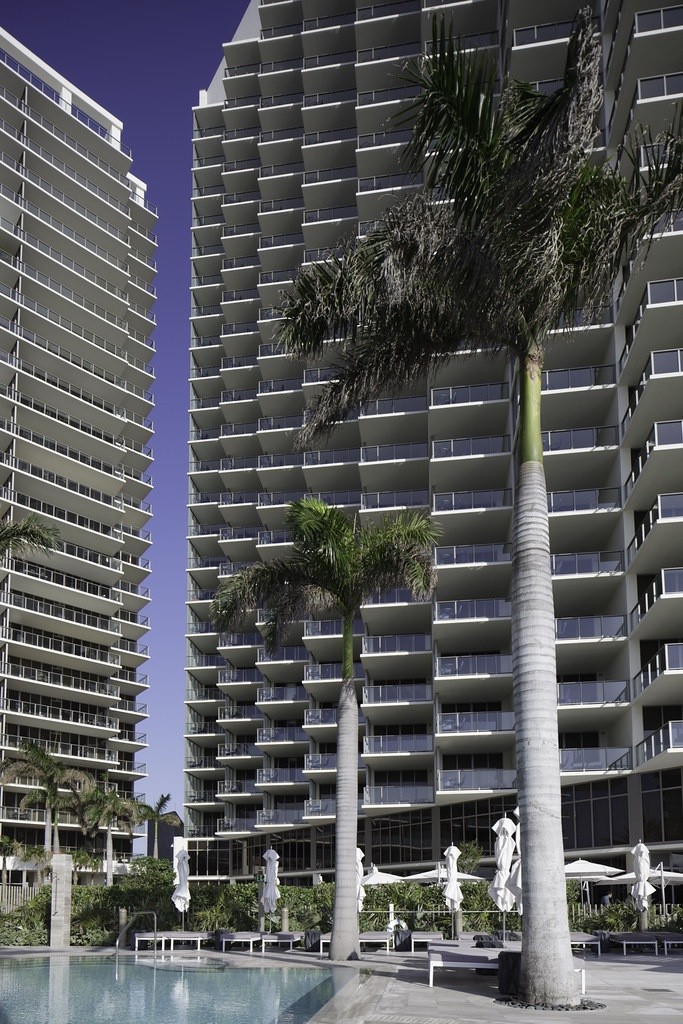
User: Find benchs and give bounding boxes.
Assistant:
[135,931,683,995]
